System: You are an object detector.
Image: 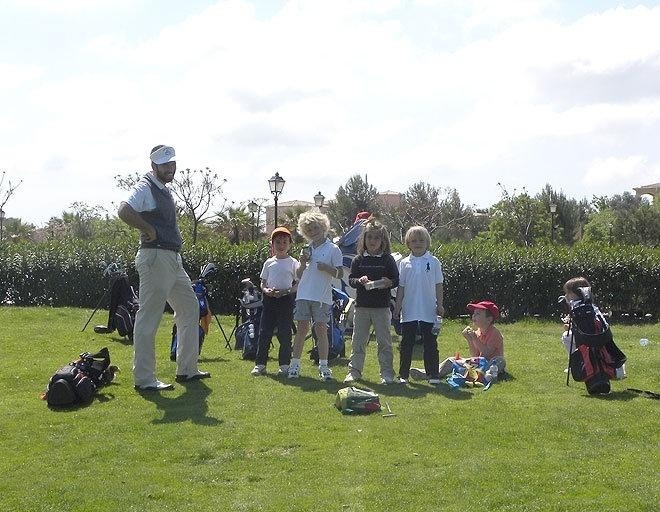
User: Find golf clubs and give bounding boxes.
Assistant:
[382,399,397,418]
[199,262,232,351]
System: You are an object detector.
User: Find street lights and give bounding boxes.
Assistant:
[268,170,285,229]
[609,223,613,247]
[248,199,259,241]
[548,201,558,244]
[313,187,326,208]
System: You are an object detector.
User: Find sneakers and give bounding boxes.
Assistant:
[174,371,211,381]
[250,365,498,388]
[134,379,173,390]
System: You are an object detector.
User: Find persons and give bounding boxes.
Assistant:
[116,143,211,390]
[287,208,344,379]
[332,212,370,332]
[344,217,400,385]
[558,275,629,380]
[249,225,300,378]
[408,298,507,381]
[393,225,445,382]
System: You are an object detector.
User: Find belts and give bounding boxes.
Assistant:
[138,243,181,252]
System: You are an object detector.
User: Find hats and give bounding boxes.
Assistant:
[467,301,499,319]
[150,145,180,164]
[271,227,291,244]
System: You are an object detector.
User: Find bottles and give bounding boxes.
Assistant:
[364,279,386,290]
[431,314,443,335]
[490,360,499,381]
[302,246,313,266]
